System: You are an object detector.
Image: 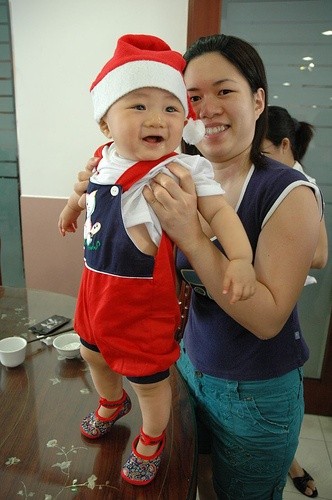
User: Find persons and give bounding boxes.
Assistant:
[75,34,323,500]
[256,106,328,498]
[58,35,256,486]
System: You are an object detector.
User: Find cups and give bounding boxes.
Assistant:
[0,337,27,367]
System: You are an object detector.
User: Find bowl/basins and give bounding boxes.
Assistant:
[53,334,81,359]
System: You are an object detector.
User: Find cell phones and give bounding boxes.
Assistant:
[31,315,71,335]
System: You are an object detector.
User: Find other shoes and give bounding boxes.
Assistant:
[122,428,168,485]
[80,389,130,437]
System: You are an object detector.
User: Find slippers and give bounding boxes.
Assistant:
[288,468,318,499]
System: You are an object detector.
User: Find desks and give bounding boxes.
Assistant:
[0,286,198,500]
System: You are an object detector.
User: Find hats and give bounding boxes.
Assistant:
[89,34,207,144]
